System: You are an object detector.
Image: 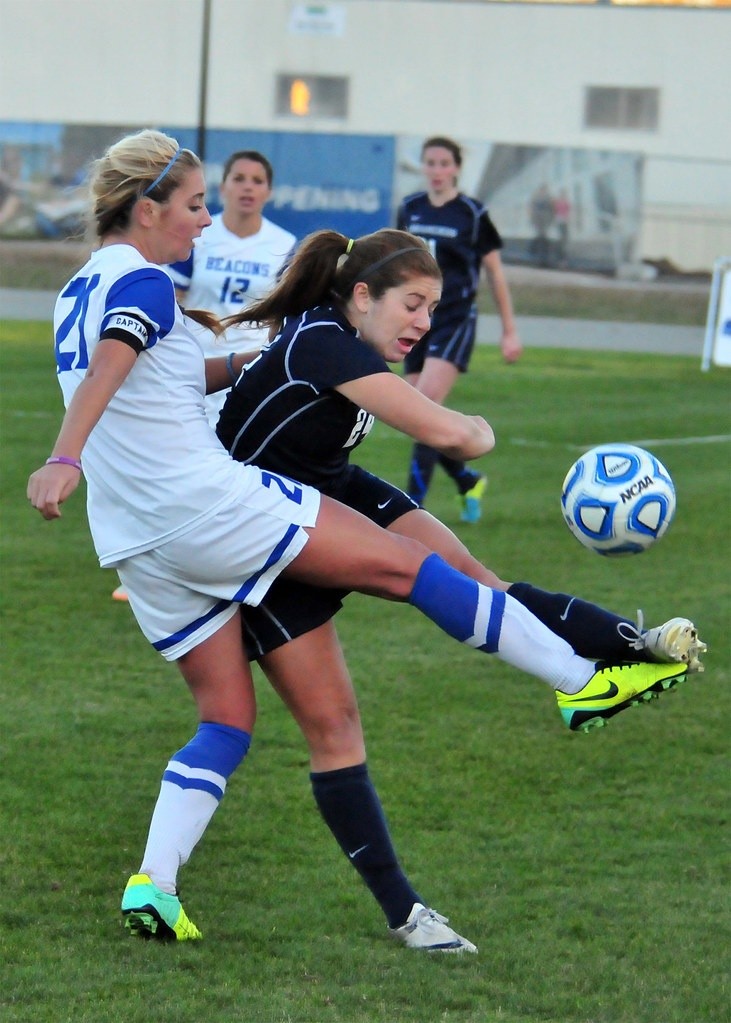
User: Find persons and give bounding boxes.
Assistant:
[214,227,709,955]
[164,150,301,429]
[26,125,691,945]
[395,135,526,525]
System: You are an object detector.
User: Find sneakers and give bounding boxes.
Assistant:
[120,872,202,942]
[554,661,687,734]
[386,902,478,952]
[457,473,487,522]
[616,607,708,674]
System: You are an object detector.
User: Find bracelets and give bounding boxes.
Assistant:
[226,352,238,381]
[46,456,80,470]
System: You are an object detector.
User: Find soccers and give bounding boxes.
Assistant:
[558,442,679,558]
